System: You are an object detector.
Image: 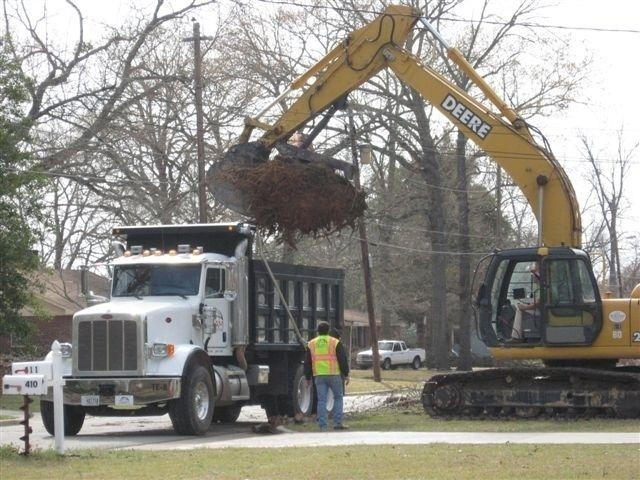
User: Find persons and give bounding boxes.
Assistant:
[305,328,344,420]
[303,322,351,430]
[505,269,541,343]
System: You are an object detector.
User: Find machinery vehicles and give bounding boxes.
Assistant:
[206,4,640,423]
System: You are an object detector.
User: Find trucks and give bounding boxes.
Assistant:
[40,221,345,437]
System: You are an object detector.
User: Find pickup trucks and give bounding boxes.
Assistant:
[356,340,426,370]
